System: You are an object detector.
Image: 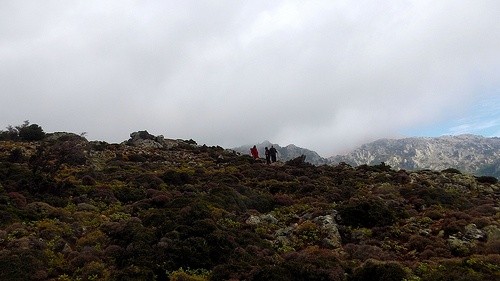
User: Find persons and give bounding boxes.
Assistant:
[268,146,278,163]
[251,145,257,159]
[250,148,259,160]
[264,147,271,164]
